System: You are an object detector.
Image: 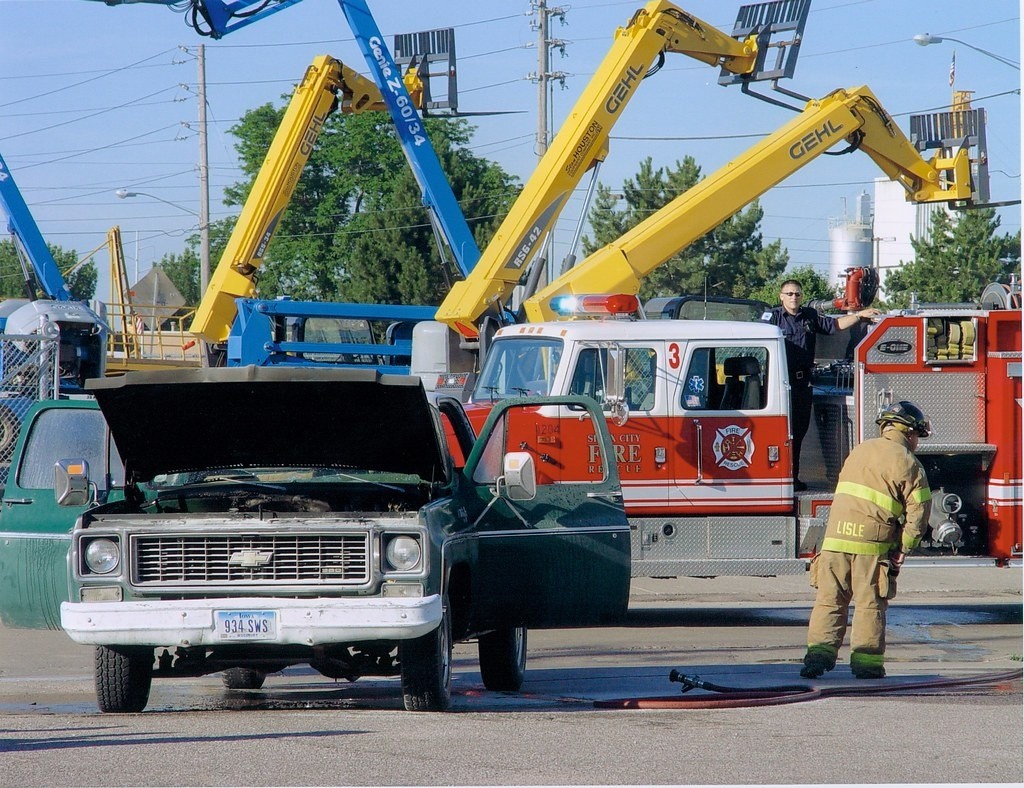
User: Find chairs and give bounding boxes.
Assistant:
[719,356,760,410]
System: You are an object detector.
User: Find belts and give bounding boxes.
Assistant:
[790,370,810,380]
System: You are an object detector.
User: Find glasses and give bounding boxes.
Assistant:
[782,291,801,297]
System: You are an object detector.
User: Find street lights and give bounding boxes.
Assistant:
[113,188,211,302]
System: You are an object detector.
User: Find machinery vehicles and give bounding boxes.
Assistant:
[155,0,994,410]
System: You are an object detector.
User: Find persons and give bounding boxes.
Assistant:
[758,279,881,492]
[799,400,932,680]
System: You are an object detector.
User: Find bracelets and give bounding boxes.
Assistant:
[855,310,860,319]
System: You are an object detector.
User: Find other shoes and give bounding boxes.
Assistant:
[800,665,818,679]
[856,666,884,678]
[794,480,808,492]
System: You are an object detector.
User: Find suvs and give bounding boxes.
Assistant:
[0,367,637,716]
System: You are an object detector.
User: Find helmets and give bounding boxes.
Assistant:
[875,401,934,439]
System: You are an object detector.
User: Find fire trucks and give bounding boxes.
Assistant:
[434,292,1022,582]
